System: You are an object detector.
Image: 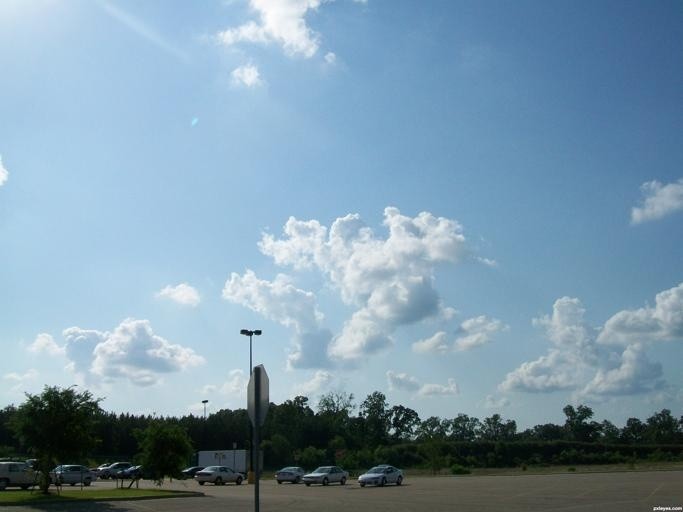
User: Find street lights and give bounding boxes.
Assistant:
[202,400,208,419]
[240,329,261,471]
[233,443,236,471]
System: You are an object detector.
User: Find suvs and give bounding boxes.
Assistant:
[0,461,46,491]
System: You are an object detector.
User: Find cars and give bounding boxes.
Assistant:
[275,467,306,483]
[175,466,245,485]
[301,466,349,486]
[48,464,97,486]
[90,462,145,479]
[358,465,404,487]
